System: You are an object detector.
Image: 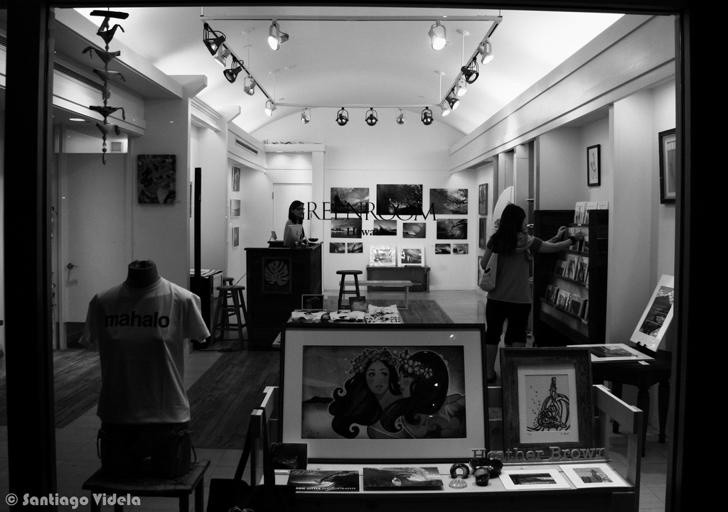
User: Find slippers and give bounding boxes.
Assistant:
[487,370,496,383]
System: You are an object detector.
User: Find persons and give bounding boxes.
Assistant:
[283,198,310,246]
[326,348,407,440]
[380,351,466,439]
[81,261,214,485]
[479,203,589,387]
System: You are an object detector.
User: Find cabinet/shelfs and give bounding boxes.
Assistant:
[537,208,589,331]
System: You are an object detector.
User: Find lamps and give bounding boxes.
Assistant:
[428,20,448,53]
[437,39,491,119]
[201,20,273,123]
[298,106,436,128]
[266,19,291,52]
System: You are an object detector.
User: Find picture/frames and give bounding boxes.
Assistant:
[658,128,678,204]
[278,320,583,463]
[585,143,601,188]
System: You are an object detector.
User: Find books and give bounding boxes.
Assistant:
[545,202,608,322]
[287,468,360,492]
[362,465,444,491]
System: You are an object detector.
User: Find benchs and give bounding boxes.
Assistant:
[338,280,412,311]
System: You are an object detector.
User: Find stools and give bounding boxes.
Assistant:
[209,277,245,343]
[336,270,362,310]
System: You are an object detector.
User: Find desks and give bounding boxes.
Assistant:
[587,348,671,446]
[366,265,431,294]
[82,457,210,512]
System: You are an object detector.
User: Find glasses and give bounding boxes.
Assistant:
[296,207,305,211]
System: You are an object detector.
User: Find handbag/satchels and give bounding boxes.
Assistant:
[207,477,297,512]
[479,253,498,291]
